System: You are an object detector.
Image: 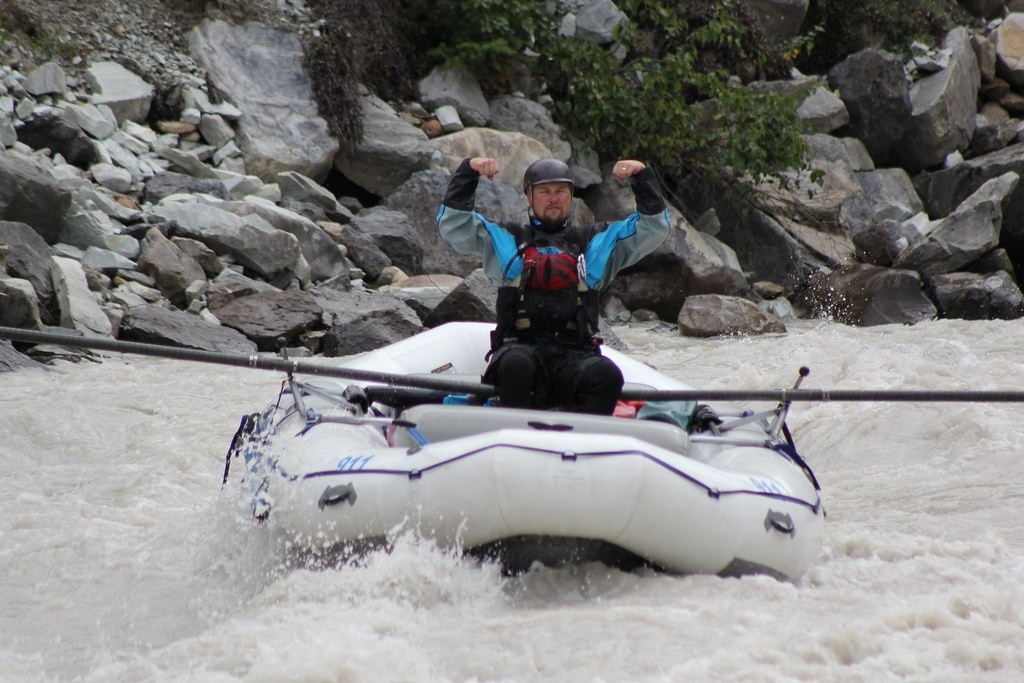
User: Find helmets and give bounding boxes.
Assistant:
[523,159,574,194]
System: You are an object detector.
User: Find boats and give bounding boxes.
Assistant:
[222,317,831,585]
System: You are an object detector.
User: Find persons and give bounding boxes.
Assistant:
[437,155,672,427]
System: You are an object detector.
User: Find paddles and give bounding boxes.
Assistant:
[617,389,1024,403]
[0,322,509,399]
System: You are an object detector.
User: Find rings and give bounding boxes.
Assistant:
[620,168,627,171]
